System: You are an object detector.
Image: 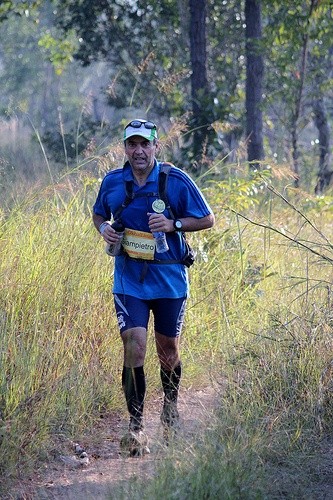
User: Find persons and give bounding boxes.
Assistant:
[91,119,215,456]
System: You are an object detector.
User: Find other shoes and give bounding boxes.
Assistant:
[120,430,151,458]
[160,409,179,444]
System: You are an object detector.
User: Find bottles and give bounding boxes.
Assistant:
[150,213,169,254]
[105,218,125,257]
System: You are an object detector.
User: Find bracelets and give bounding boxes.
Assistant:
[99,222,111,235]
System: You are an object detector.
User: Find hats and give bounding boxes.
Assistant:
[122,119,157,143]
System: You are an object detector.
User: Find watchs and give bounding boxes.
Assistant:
[173,219,184,232]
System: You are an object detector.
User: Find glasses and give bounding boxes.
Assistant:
[124,120,156,130]
